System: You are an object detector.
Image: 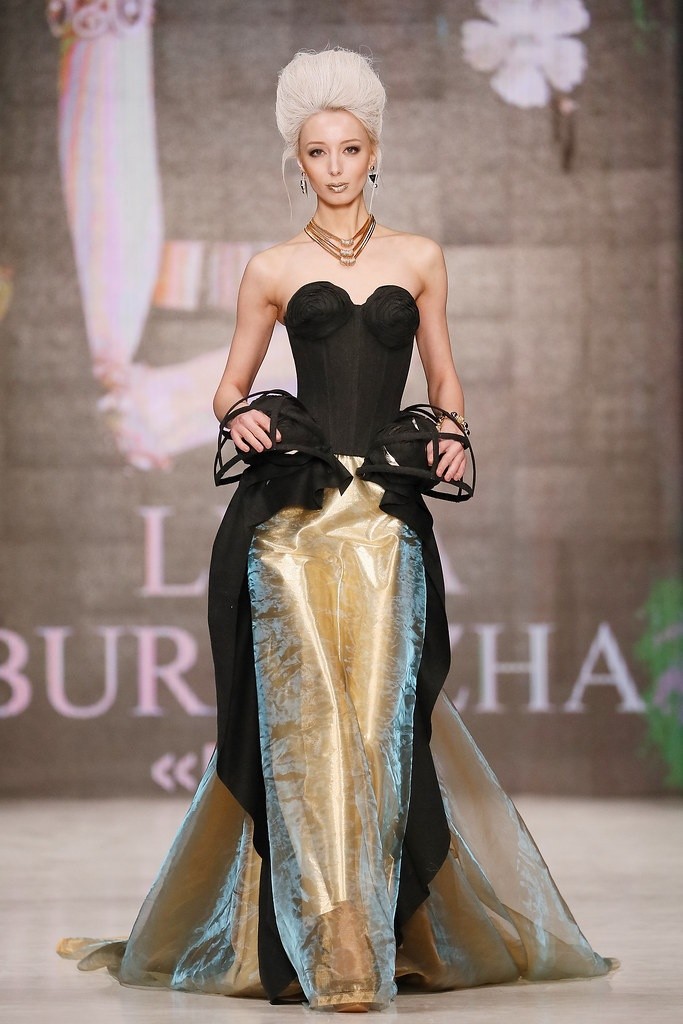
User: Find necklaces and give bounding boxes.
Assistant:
[304,214,376,266]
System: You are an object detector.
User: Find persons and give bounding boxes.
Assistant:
[57,50,619,1011]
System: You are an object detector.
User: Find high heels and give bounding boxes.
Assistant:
[330,977,373,1011]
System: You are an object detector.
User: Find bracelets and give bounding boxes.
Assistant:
[436,412,470,437]
